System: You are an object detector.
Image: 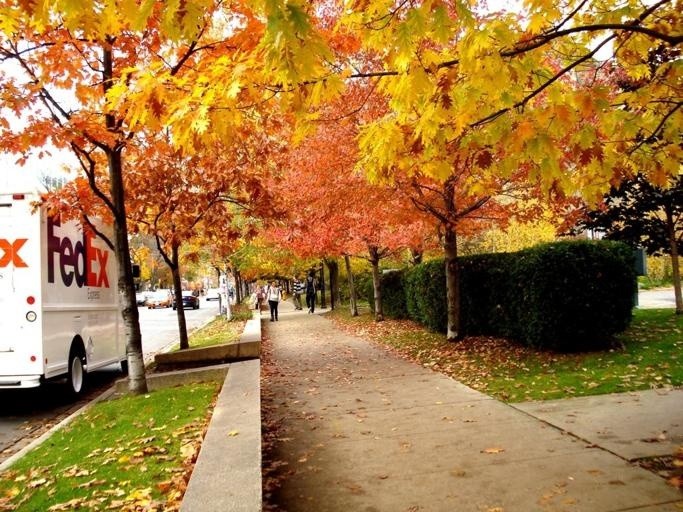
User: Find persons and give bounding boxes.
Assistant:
[266,281,281,322]
[290,274,303,310]
[303,271,319,314]
[254,283,282,310]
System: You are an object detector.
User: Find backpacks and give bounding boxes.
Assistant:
[306,279,314,297]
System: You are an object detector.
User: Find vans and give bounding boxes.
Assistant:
[0,193,139,399]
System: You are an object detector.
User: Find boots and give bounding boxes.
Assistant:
[270,315,278,321]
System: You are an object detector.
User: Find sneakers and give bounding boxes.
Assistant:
[308,307,312,315]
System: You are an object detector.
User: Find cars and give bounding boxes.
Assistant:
[206,282,236,301]
[135,289,200,310]
